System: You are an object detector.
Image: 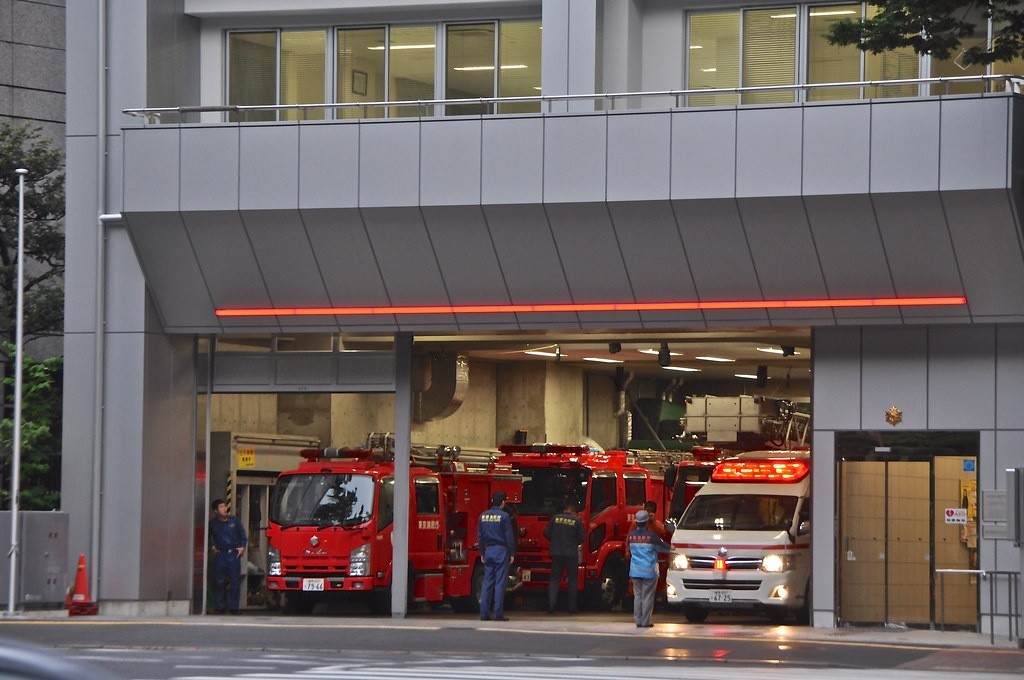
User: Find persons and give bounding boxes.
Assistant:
[625,501,670,558]
[477,491,520,621]
[627,511,670,628]
[206,499,248,616]
[542,501,586,615]
[963,489,968,514]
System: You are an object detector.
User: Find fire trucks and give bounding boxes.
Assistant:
[263,439,523,612]
[496,442,690,612]
[664,445,735,536]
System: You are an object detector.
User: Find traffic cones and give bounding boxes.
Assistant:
[64,554,99,615]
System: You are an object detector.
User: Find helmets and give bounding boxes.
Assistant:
[634,510,649,522]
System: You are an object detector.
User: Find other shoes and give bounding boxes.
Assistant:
[569,611,580,614]
[549,611,559,614]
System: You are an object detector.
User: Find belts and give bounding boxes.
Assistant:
[486,541,504,546]
[216,547,237,554]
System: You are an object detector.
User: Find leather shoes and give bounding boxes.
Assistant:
[637,624,654,627]
[230,609,242,615]
[217,610,226,615]
[493,617,510,621]
[480,617,493,620]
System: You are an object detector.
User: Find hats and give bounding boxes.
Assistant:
[493,491,505,498]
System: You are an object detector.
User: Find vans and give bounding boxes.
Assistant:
[663,451,815,623]
